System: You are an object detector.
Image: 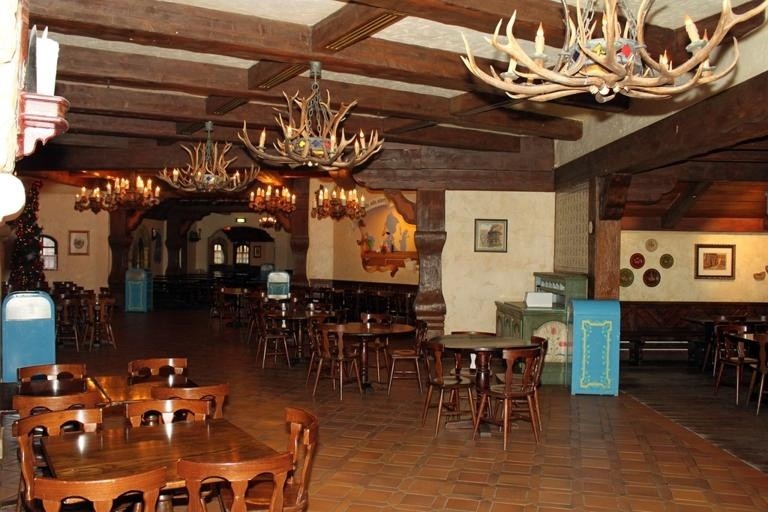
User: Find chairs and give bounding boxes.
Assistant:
[10,278,119,351]
[684,314,767,415]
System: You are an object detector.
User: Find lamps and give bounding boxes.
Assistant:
[459,0,767,103]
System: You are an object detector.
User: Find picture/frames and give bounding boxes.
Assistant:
[473,218,508,253]
[68,230,89,256]
[694,244,736,280]
[253,245,262,258]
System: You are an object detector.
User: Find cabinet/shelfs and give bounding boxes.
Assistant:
[494,272,588,385]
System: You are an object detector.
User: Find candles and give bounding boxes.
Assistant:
[173,168,178,183]
[35,27,59,97]
[236,170,241,184]
